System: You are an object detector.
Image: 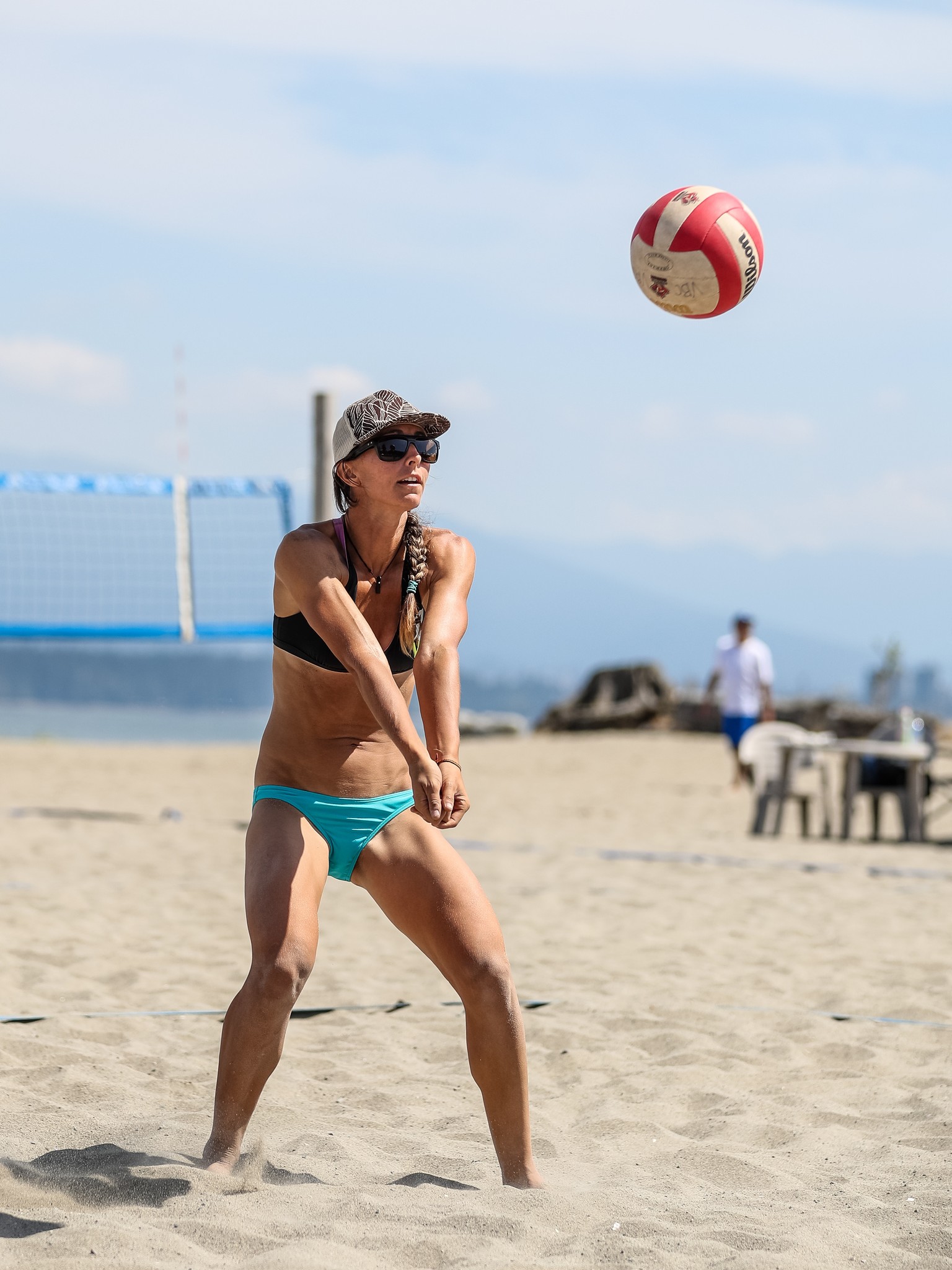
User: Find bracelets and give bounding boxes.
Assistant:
[434,752,462,772]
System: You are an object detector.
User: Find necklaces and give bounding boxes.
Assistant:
[343,513,404,594]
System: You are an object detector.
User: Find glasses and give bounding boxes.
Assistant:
[348,435,443,464]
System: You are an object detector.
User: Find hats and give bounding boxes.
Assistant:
[332,389,450,463]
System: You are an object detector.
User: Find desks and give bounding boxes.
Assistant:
[773,739,931,842]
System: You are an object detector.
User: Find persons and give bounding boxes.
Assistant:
[703,612,774,788]
[203,390,540,1192]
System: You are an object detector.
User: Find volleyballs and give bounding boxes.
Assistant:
[629,185,765,320]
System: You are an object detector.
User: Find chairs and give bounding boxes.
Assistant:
[836,706,940,840]
[738,720,833,841]
